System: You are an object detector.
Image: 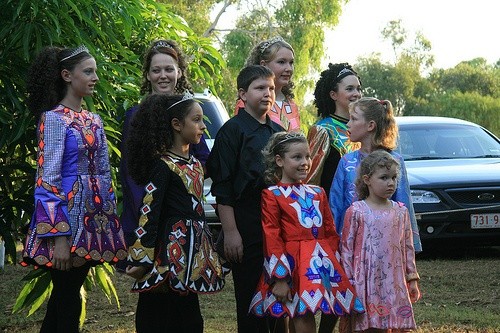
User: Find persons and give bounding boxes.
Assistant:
[206,65,287,333]
[120,40,212,247]
[117,103,225,333]
[316,97,422,333]
[27,44,128,333]
[338,149,421,333]
[247,130,367,333]
[302,61,361,204]
[233,37,300,133]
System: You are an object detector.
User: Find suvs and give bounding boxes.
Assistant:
[191,88,231,226]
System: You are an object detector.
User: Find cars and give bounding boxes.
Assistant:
[394,115,500,257]
